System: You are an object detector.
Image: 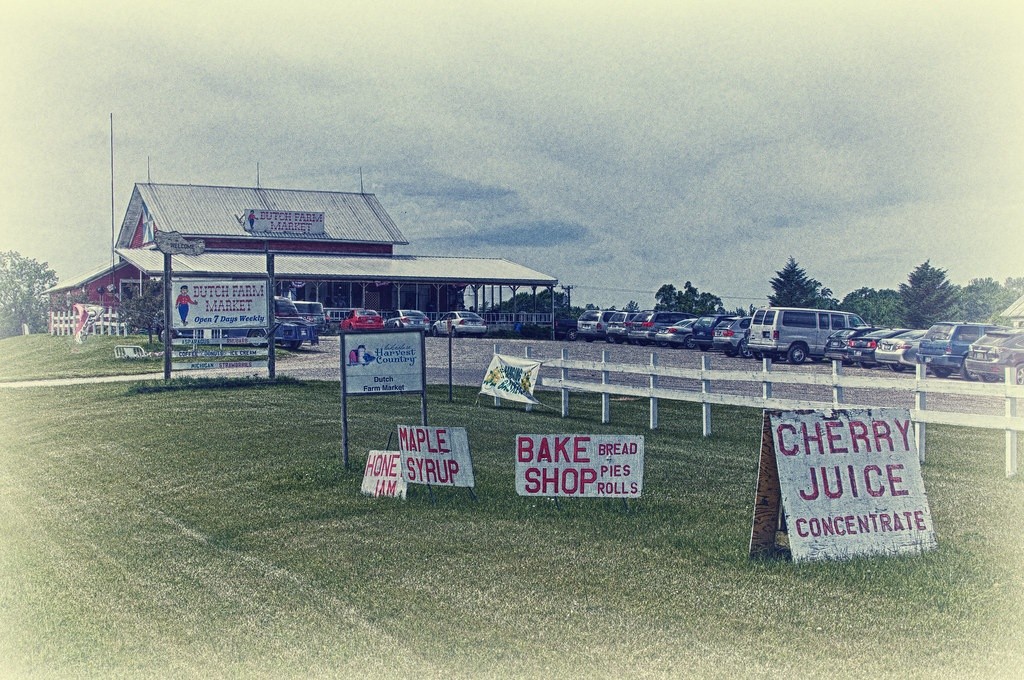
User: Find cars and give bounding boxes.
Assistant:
[382,309,432,336]
[156,296,320,348]
[655,320,699,349]
[341,311,383,332]
[847,326,913,369]
[825,328,876,368]
[432,312,489,340]
[873,329,928,374]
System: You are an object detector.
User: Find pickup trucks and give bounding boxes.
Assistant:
[553,319,579,342]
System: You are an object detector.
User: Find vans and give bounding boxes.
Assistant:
[746,306,874,364]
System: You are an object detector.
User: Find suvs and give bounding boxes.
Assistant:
[964,328,1024,386]
[605,310,642,344]
[576,309,619,343]
[713,316,756,357]
[691,315,731,353]
[294,301,329,332]
[628,309,699,348]
[916,322,1008,381]
